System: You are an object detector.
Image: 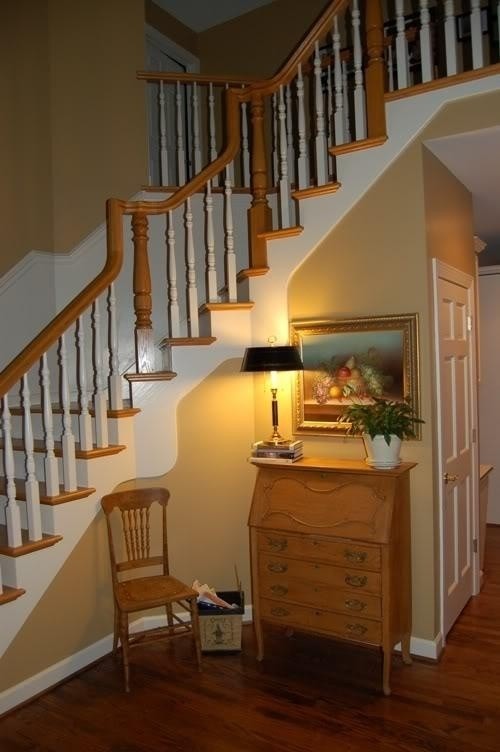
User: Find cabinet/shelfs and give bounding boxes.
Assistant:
[247,457,418,696]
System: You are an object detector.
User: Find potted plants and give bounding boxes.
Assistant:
[336,396,425,470]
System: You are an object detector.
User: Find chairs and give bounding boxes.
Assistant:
[100,486,204,693]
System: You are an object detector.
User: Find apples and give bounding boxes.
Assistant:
[338,366,352,378]
[351,367,361,378]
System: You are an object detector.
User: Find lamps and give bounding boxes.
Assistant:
[239,336,305,446]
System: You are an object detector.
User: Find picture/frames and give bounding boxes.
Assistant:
[290,312,421,441]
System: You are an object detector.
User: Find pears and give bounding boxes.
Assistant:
[346,355,356,369]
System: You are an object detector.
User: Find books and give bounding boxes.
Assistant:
[249,439,304,464]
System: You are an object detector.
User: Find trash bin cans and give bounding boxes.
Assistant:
[197,590,245,651]
